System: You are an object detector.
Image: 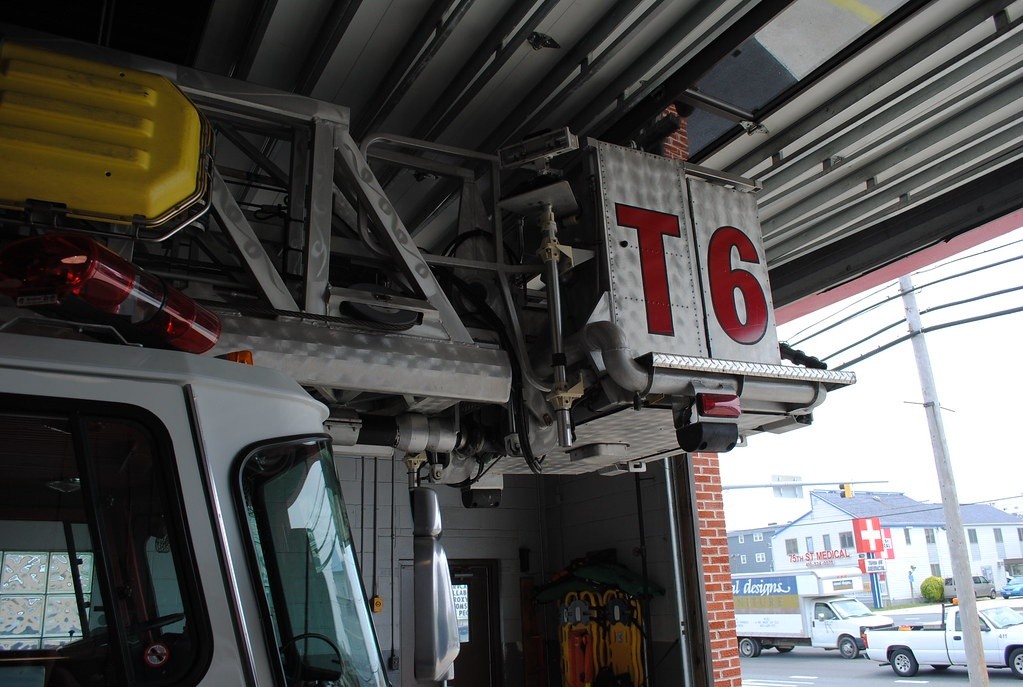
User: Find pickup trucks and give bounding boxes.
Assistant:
[862,599,1023,681]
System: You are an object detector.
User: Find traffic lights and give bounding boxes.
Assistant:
[839,483,852,499]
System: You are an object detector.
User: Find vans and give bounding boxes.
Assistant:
[943,575,996,603]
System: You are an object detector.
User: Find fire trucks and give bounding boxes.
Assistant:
[0,32,857,687]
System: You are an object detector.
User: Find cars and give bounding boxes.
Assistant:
[1000,576,1023,599]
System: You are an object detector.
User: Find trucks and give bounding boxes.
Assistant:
[731,567,894,660]
